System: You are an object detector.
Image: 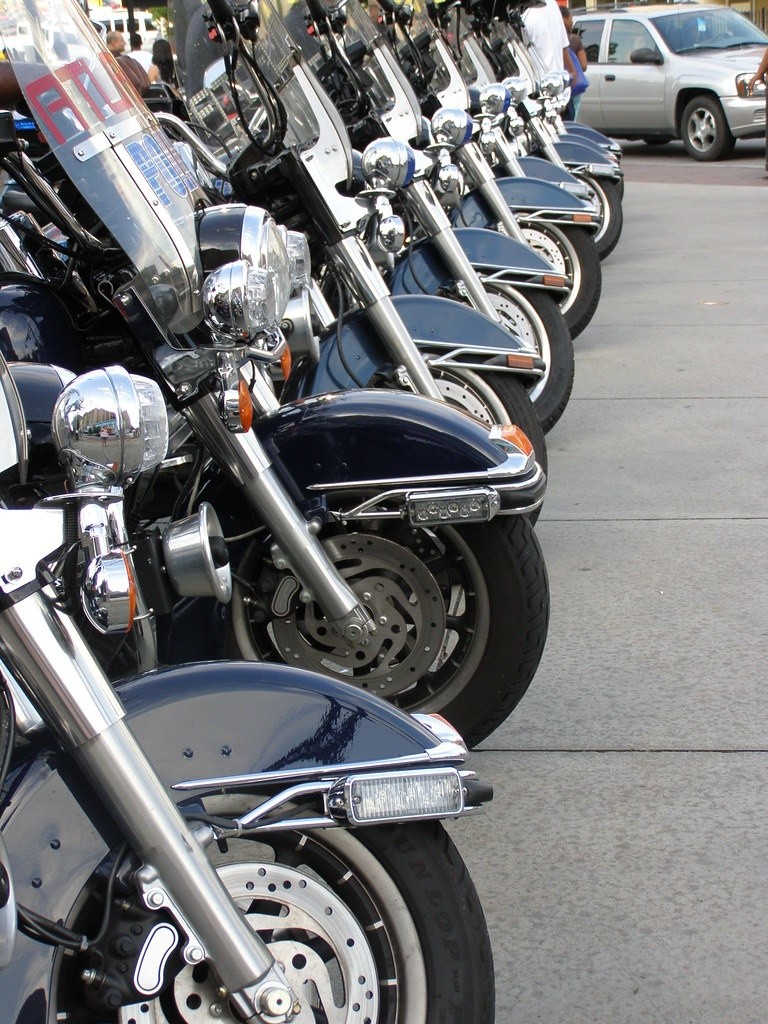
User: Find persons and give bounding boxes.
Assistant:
[521,0,587,122]
[749,47,768,180]
[107,30,176,88]
[625,18,733,64]
[0,54,152,116]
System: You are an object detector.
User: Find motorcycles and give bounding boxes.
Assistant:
[0,0,629,1023]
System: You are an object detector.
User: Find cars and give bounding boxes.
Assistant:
[565,2,768,163]
